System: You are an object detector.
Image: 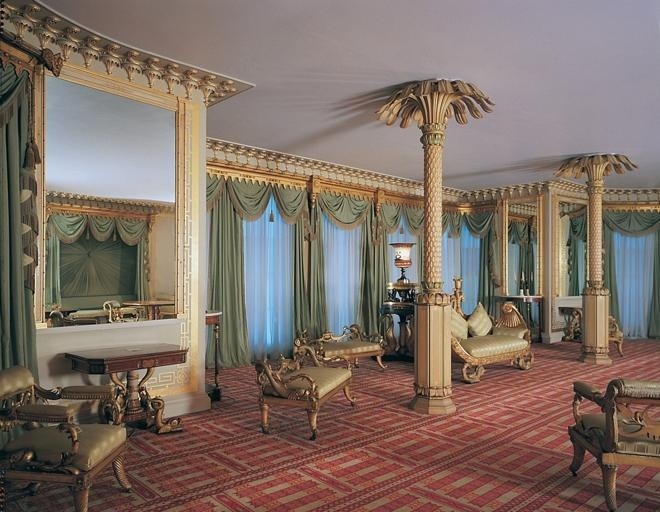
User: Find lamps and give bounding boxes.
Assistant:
[389,241,416,283]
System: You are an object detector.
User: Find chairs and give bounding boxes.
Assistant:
[1,365,131,510]
[565,377,660,510]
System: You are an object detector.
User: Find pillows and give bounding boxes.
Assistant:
[468,303,492,336]
[452,306,468,340]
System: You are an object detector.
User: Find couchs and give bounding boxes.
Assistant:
[449,302,534,383]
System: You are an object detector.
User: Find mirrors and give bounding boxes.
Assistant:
[36,67,187,326]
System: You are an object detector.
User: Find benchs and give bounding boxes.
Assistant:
[292,324,387,372]
[257,336,356,440]
[573,309,625,356]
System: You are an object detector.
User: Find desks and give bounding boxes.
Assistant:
[65,342,189,435]
